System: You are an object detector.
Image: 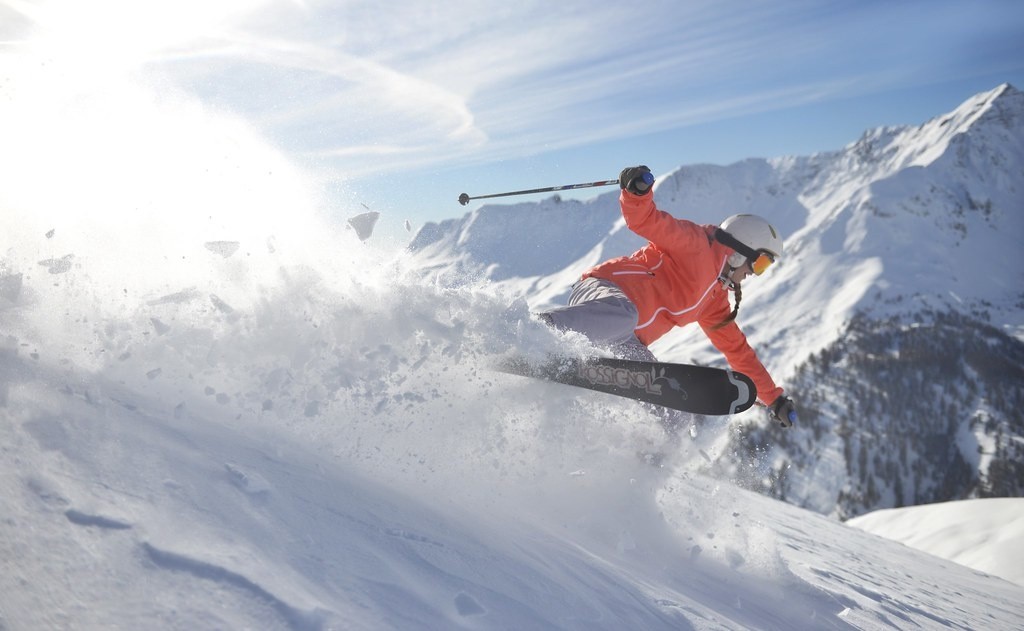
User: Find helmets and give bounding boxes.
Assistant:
[719,212,784,268]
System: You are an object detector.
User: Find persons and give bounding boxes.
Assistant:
[529,164,795,431]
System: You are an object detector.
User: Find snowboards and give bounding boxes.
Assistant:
[484,354,757,416]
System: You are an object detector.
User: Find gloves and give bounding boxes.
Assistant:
[769,395,795,428]
[619,165,654,195]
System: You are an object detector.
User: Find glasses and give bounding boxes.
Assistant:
[714,228,775,276]
